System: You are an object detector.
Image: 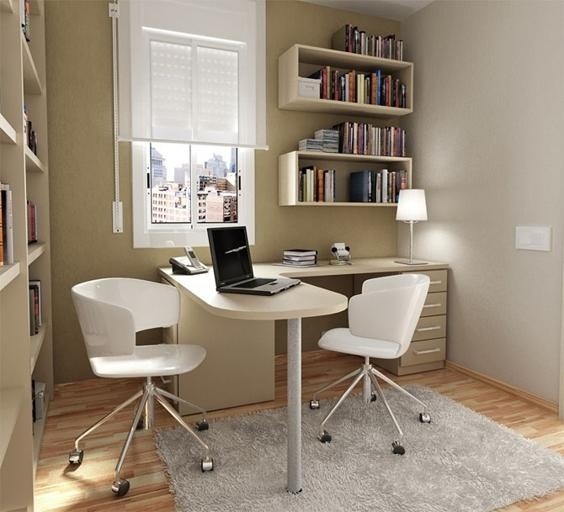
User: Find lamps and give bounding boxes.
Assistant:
[394,189,428,267]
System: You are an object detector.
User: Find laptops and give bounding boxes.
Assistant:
[207,226,301,296]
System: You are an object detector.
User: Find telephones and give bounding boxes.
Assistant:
[169,246,208,275]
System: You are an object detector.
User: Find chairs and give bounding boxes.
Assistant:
[69,277,216,497]
[308,273,430,456]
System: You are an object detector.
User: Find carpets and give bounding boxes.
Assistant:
[150,384,564,512]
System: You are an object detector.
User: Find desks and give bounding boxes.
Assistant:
[156,248,450,418]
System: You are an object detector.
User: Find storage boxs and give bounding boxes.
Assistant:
[297,76,322,99]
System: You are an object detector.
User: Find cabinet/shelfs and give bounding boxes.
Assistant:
[277,149,414,206]
[278,43,414,118]
[1,0,54,512]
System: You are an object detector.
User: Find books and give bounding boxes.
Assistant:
[280,247,317,266]
[22,104,38,159]
[28,279,43,336]
[296,20,411,206]
[25,200,38,244]
[0,183,14,269]
[19,1,34,43]
